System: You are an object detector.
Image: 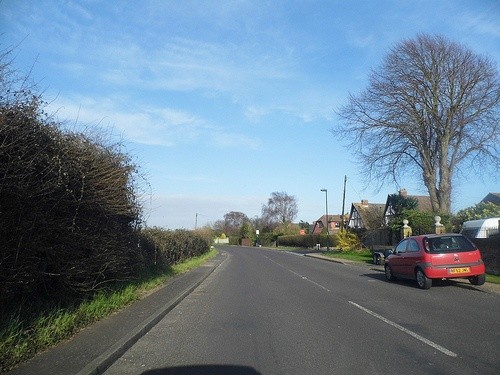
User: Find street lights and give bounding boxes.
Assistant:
[320,188,330,250]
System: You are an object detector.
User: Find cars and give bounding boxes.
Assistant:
[385,232,487,290]
